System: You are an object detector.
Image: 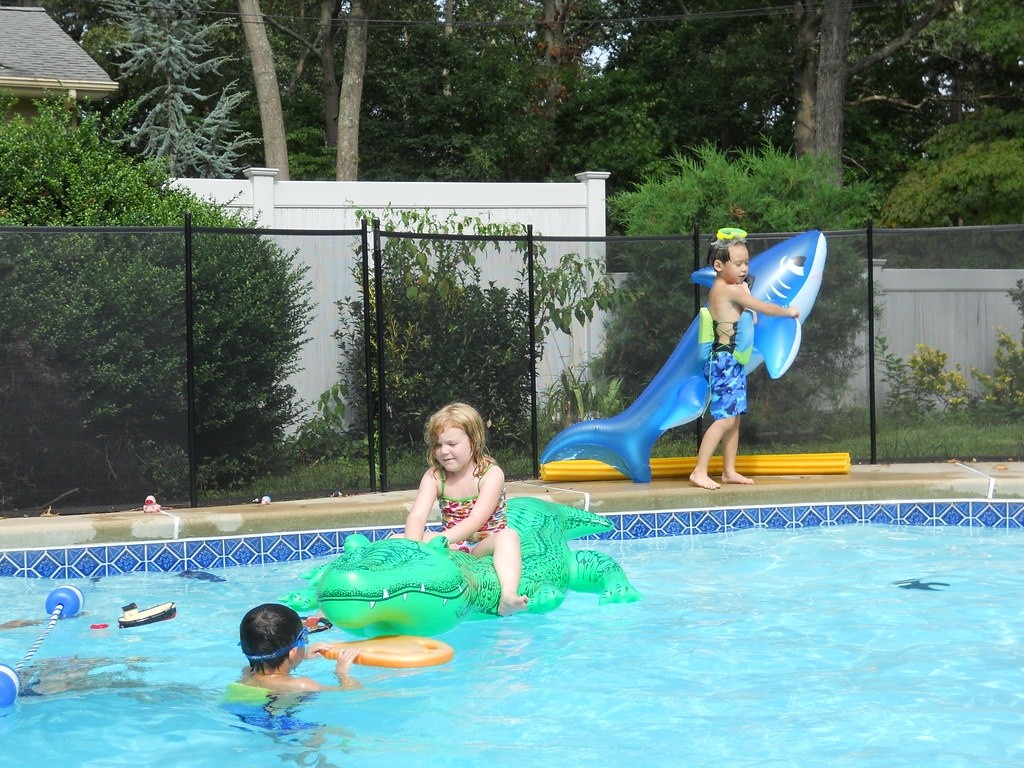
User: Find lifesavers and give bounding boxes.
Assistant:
[539,449,852,475]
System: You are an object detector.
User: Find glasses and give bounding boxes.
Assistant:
[296,627,310,647]
[716,228,748,240]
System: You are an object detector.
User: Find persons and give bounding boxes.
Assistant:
[236,603,361,691]
[389,403,529,615]
[689,228,800,490]
